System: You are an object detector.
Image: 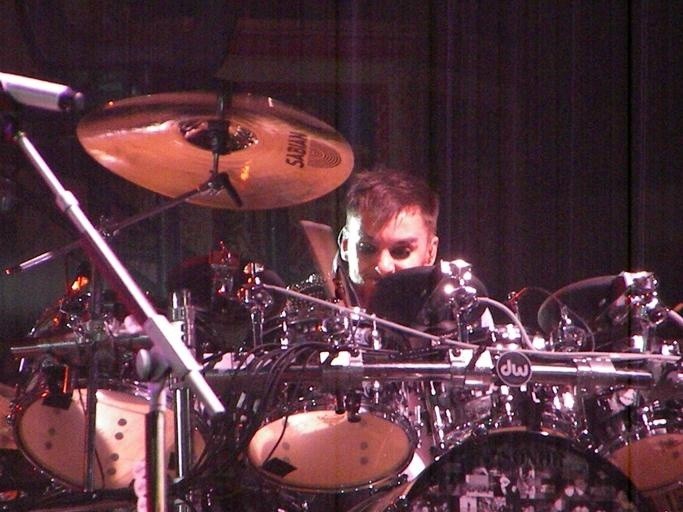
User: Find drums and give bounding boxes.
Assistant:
[552,334,683,497]
[1,449,181,511]
[6,289,213,492]
[367,425,654,510]
[244,326,423,493]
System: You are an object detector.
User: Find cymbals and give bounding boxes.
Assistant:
[76,87,354,211]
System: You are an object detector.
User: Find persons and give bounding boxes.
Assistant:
[340,169,440,345]
